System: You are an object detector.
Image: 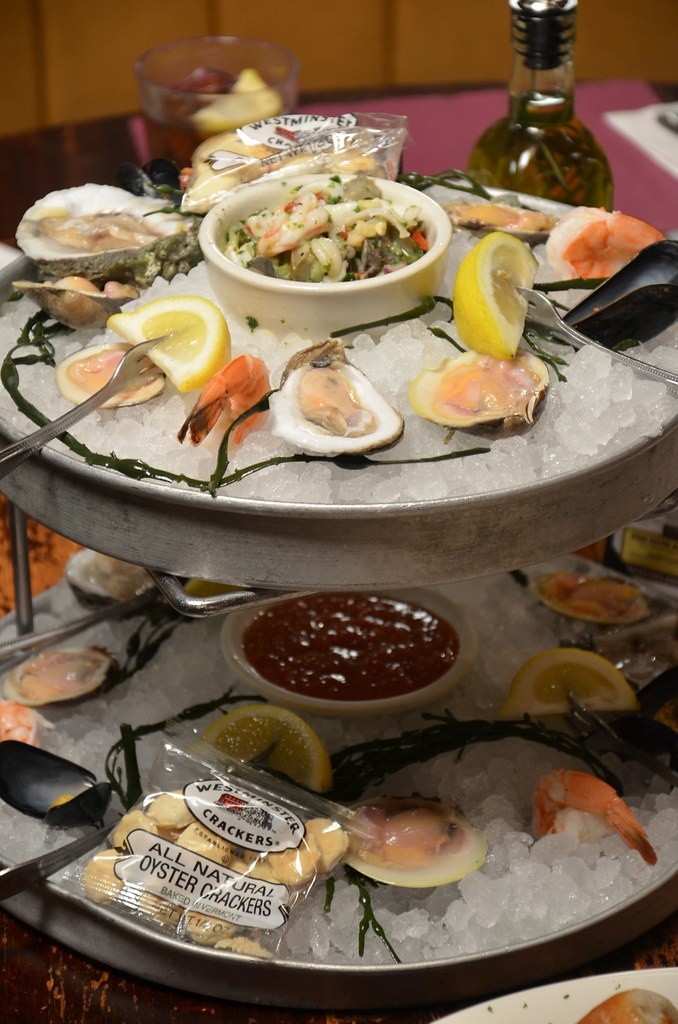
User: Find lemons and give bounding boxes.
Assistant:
[190,67,283,133]
[449,230,539,363]
[192,705,330,801]
[101,295,234,398]
[181,578,239,597]
[498,643,641,718]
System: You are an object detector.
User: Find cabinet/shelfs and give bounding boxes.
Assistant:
[0,0,676,193]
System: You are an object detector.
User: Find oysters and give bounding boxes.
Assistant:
[3,645,125,706]
[62,548,159,608]
[331,792,494,889]
[443,200,562,245]
[15,183,199,290]
[269,341,406,457]
[530,570,649,627]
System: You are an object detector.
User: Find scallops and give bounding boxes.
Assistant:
[120,158,184,204]
[417,349,553,438]
[63,341,167,411]
[0,742,112,824]
[555,241,678,353]
[13,275,141,327]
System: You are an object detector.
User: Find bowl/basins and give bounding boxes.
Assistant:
[221,586,472,716]
[198,169,453,346]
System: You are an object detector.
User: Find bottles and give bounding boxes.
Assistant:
[466,1,614,210]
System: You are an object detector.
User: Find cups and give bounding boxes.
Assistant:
[132,35,301,189]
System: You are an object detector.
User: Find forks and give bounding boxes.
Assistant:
[515,286,677,399]
[1,331,168,485]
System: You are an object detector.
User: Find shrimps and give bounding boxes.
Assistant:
[529,766,657,869]
[547,205,668,280]
[178,356,269,446]
